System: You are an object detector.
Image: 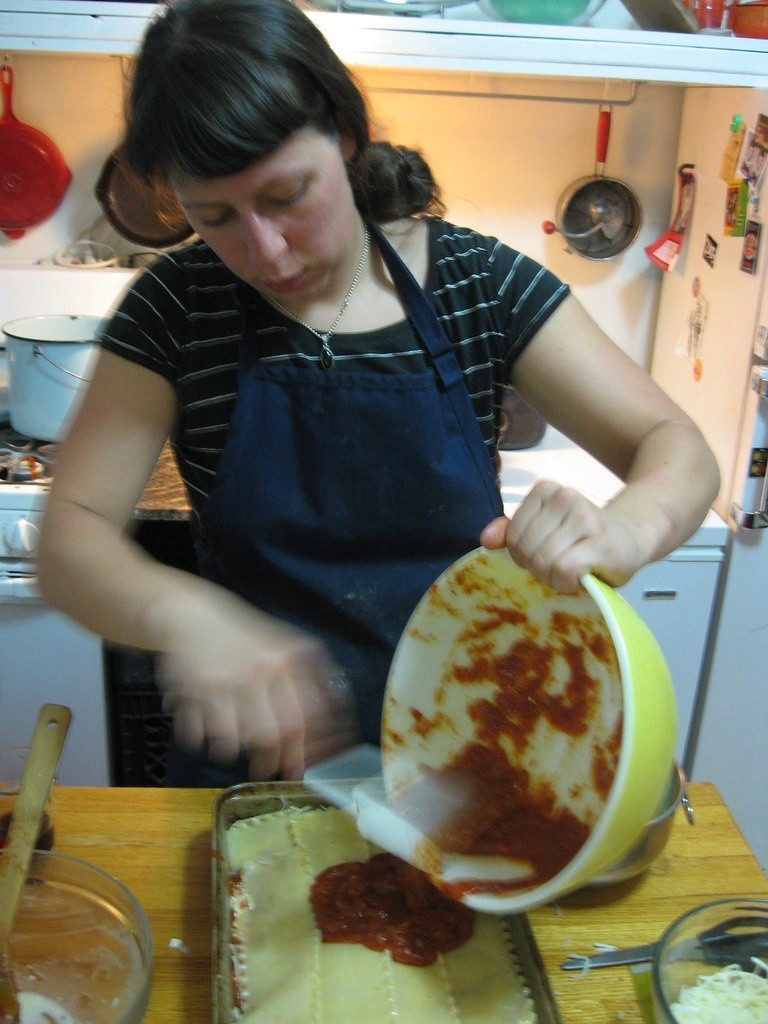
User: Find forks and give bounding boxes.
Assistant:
[303,773,443,876]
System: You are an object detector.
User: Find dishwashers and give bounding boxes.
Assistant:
[0,511,113,789]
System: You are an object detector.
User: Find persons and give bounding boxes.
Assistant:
[37,0,721,783]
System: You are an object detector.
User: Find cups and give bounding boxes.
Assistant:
[0,746,58,885]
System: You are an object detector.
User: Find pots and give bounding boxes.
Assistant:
[92,137,197,249]
[543,103,644,263]
[0,64,73,241]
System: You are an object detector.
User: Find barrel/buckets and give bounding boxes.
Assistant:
[1,315,110,445]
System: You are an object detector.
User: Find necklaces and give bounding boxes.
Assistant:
[266,224,371,368]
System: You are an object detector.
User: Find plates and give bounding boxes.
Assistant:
[210,779,563,1024]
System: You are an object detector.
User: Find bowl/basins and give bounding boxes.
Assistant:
[380,544,678,914]
[580,756,695,889]
[649,896,768,1023]
[721,0,768,40]
[1,850,153,1024]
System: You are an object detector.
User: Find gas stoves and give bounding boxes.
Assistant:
[0,401,65,510]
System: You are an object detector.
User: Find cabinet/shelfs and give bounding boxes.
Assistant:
[0,546,729,790]
